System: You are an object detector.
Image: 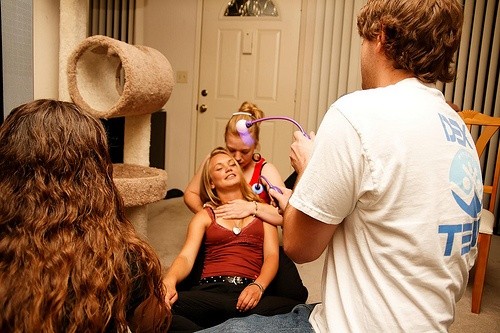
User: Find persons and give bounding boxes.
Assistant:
[193,0,484,333]
[162,146,305,333]
[176,102,308,303]
[0,99,199,333]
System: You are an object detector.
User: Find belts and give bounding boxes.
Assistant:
[201,275,256,286]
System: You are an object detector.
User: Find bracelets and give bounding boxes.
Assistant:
[249,283,264,293]
[253,200,258,217]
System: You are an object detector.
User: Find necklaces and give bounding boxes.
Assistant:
[232,218,241,235]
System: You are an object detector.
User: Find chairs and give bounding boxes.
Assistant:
[452,102,500,313]
